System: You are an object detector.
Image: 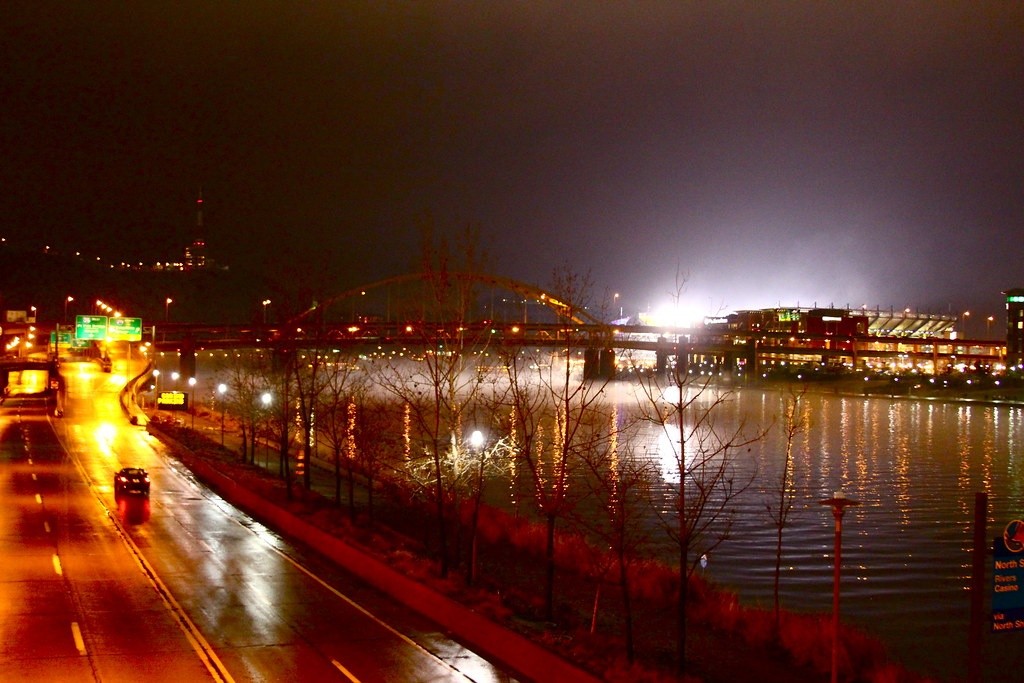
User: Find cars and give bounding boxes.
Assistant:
[113,467,151,496]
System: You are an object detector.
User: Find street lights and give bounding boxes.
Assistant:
[31,306,36,317]
[820,490,861,683]
[219,383,226,445]
[153,370,160,414]
[262,393,272,471]
[166,298,172,322]
[263,300,272,331]
[172,372,180,390]
[188,377,196,428]
[470,430,483,571]
[65,296,73,323]
